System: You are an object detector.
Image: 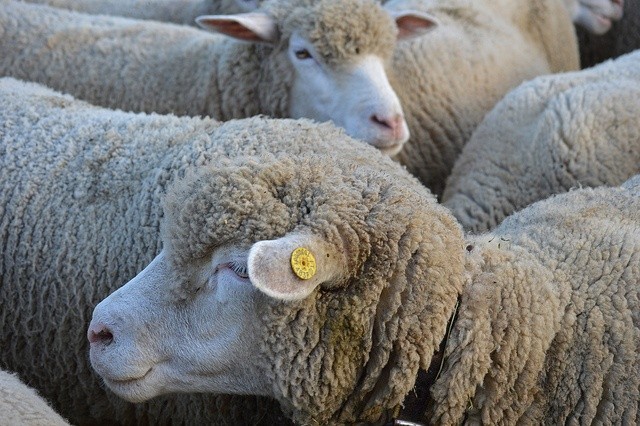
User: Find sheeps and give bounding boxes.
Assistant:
[25,0,249,30]
[0,369,71,426]
[0,0,410,158]
[88,114,639,426]
[440,50,640,233]
[0,77,295,426]
[383,1,625,202]
